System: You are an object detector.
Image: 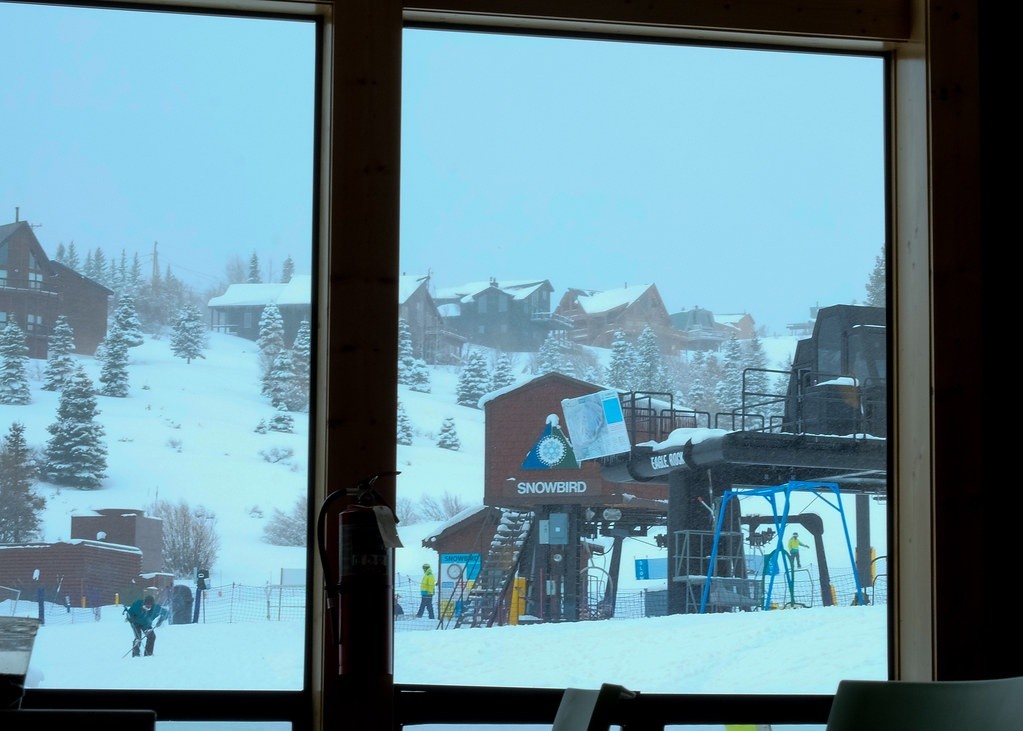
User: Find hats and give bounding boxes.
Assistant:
[422,563,430,568]
[144,595,154,606]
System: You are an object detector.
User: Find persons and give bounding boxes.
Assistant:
[416,564,436,619]
[788,532,809,568]
[126,595,169,657]
